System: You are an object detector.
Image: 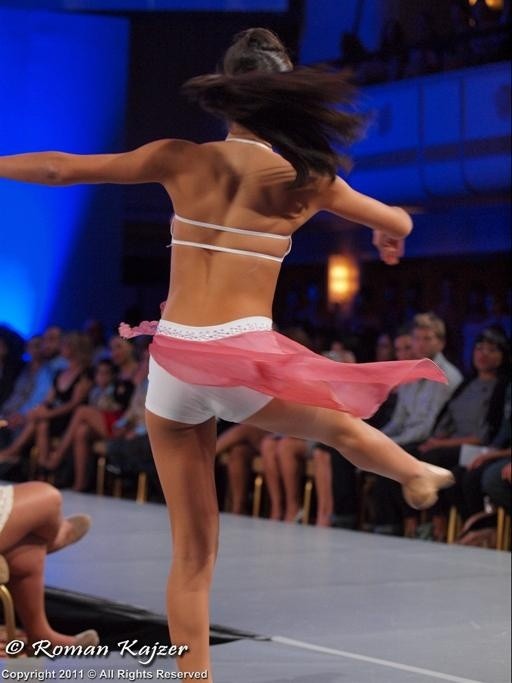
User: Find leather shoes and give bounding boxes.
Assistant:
[46,514,90,554]
[74,630,99,651]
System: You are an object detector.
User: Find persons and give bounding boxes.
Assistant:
[0,23,464,681]
[1,479,104,658]
[1,244,512,546]
[306,0,511,91]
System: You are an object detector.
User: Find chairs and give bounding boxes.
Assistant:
[24,438,512,554]
